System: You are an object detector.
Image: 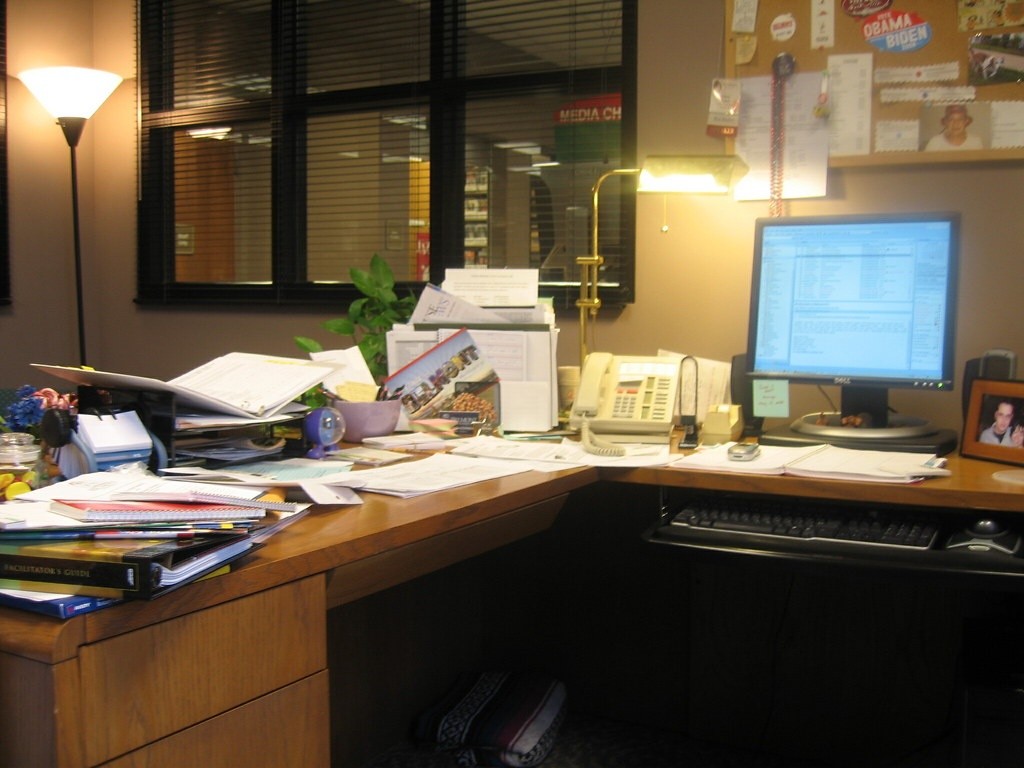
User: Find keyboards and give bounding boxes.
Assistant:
[656,492,946,559]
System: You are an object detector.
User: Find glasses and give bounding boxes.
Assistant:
[949,117,967,125]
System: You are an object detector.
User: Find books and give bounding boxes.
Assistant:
[1,299,946,623]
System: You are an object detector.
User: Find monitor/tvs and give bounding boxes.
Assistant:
[745,210,960,437]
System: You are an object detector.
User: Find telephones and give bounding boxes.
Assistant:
[568,349,681,434]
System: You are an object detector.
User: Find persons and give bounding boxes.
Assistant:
[924,106,983,151]
[981,400,1024,448]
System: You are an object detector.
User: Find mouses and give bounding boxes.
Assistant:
[973,518,999,535]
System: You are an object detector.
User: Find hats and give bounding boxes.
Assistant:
[941,104,972,127]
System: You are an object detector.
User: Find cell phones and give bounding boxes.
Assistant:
[727,443,759,462]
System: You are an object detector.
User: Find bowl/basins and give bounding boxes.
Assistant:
[334,398,403,444]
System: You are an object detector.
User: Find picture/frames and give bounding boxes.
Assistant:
[959,377,1024,468]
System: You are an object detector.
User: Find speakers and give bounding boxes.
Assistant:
[962,359,980,438]
[731,355,765,439]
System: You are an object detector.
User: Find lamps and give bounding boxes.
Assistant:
[17,64,123,369]
[572,155,750,375]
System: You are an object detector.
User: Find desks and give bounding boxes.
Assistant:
[0,436,1024,768]
